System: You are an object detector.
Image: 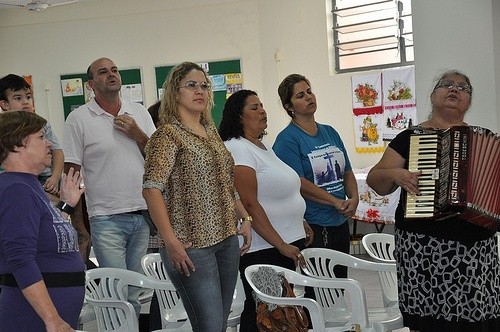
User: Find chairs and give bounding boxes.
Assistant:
[298,247,410,332]
[362,232,399,308]
[141,252,246,332]
[83,267,192,332]
[245,264,368,332]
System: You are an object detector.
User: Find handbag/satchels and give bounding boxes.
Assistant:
[255,275,309,332]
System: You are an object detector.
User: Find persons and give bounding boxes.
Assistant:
[219,89,313,332]
[144,227,180,332]
[366,72,500,332]
[271,74,359,330]
[141,62,251,332]
[0,74,65,203]
[1,110,88,332]
[62,58,157,323]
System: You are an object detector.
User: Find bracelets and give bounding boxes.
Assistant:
[303,219,306,223]
[56,200,74,215]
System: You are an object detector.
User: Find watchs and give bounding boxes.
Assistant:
[240,215,253,225]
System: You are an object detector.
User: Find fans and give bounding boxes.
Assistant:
[0,0,78,12]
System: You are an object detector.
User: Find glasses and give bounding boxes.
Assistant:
[436,82,471,93]
[178,81,210,90]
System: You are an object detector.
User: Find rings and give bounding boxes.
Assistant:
[297,254,301,258]
[121,122,124,127]
